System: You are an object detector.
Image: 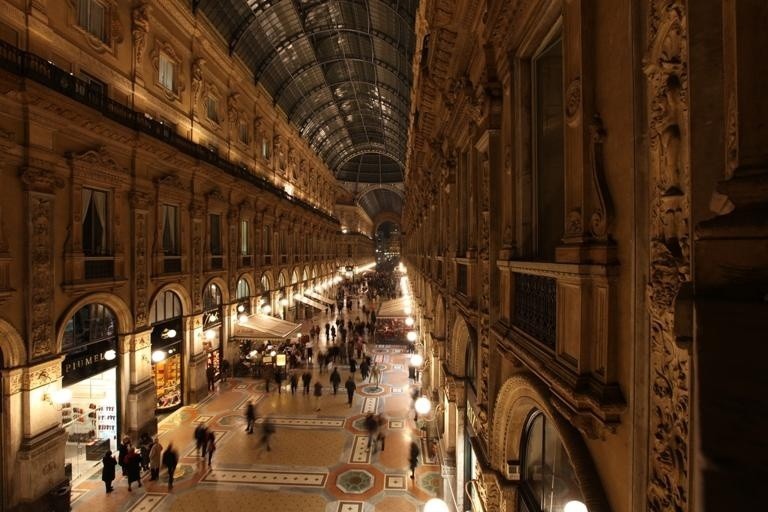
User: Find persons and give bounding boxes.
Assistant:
[244,400,255,435]
[363,411,378,452]
[408,441,419,478]
[255,412,279,452]
[260,261,397,409]
[207,363,216,391]
[371,413,389,455]
[218,357,230,383]
[100,422,218,494]
